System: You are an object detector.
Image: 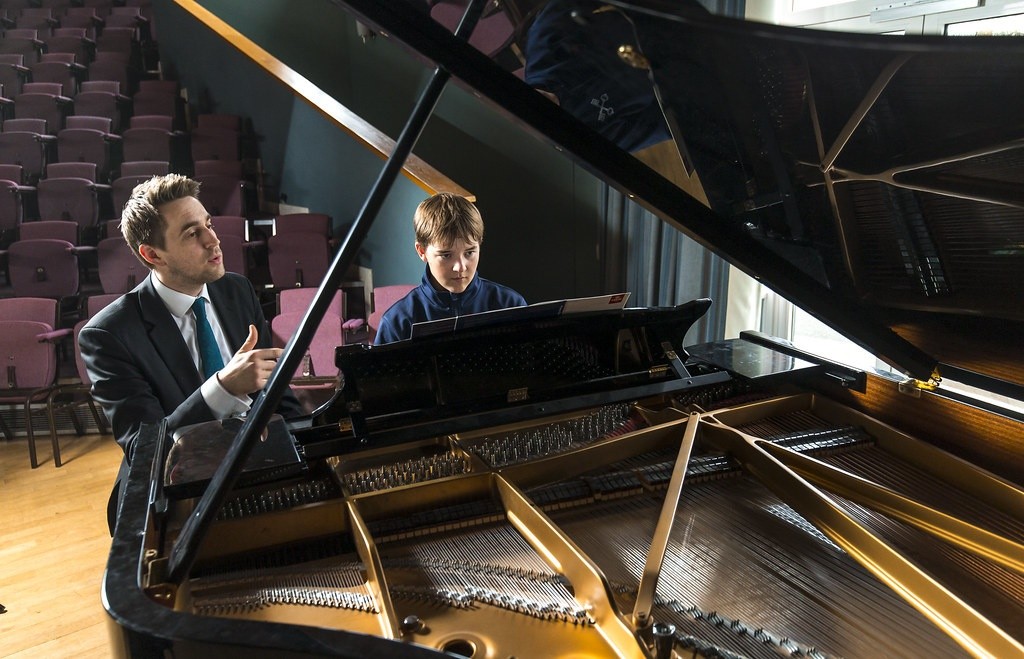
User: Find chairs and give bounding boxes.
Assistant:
[0,0,418,469]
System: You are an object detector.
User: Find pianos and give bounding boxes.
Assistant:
[101,1,1024,659]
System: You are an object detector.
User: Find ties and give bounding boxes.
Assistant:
[192,296,225,379]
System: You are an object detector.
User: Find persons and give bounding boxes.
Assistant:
[525,0,710,153]
[374,193,526,345]
[78,173,306,537]
